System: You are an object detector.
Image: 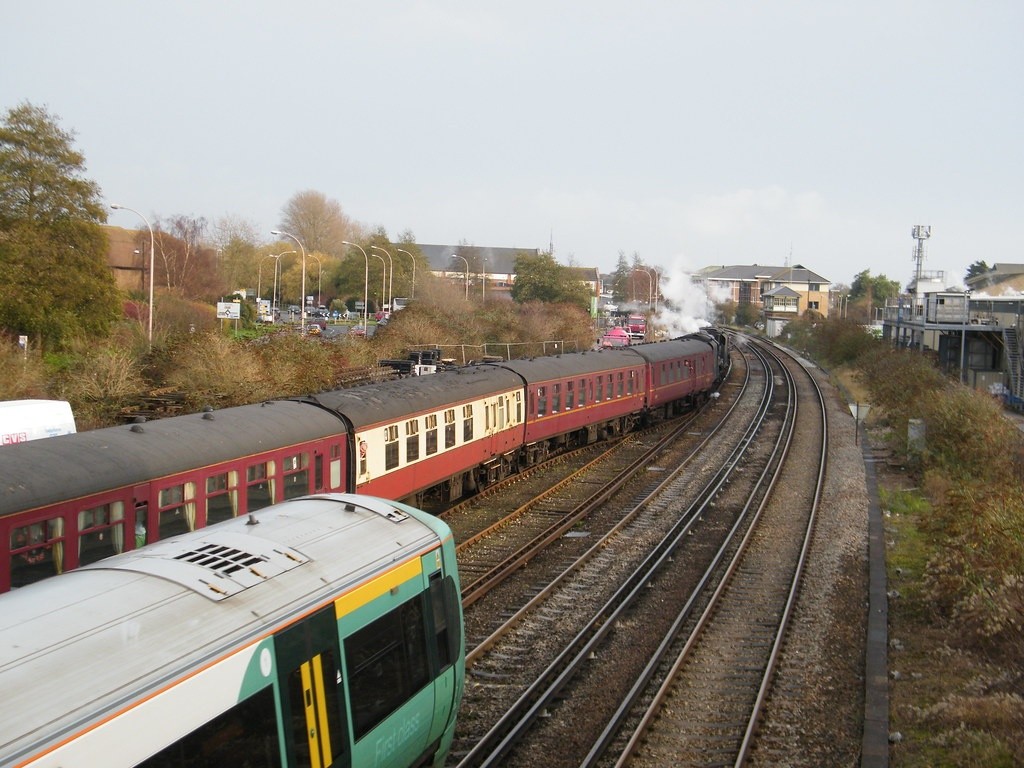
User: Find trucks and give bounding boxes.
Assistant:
[393,296,409,312]
[602,323,632,347]
[624,313,647,339]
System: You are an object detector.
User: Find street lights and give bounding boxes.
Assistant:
[109,202,489,349]
[631,257,661,315]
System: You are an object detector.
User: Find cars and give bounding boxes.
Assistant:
[374,311,389,321]
[270,306,329,336]
[347,324,366,336]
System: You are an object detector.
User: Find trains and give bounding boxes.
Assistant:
[2,492,466,768]
[1,326,731,594]
